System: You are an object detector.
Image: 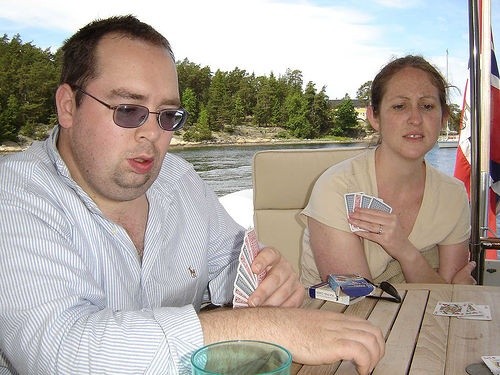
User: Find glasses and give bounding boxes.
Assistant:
[75,85,191,131]
[363,277,402,303]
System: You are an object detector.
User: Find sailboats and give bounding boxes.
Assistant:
[436,50,460,148]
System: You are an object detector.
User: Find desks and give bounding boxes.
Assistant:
[287,285,500,375]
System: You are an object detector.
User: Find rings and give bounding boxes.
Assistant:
[376,224,384,234]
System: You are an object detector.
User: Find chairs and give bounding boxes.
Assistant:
[253,147,370,280]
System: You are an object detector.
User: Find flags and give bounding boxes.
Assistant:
[452,0,500,256]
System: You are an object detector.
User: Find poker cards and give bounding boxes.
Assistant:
[465,356,500,375]
[433,301,493,321]
[232,228,266,308]
[344,192,393,232]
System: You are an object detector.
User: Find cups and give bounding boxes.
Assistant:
[190,340,292,375]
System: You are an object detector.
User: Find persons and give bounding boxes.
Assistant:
[298,53,480,286]
[0,14,386,375]
